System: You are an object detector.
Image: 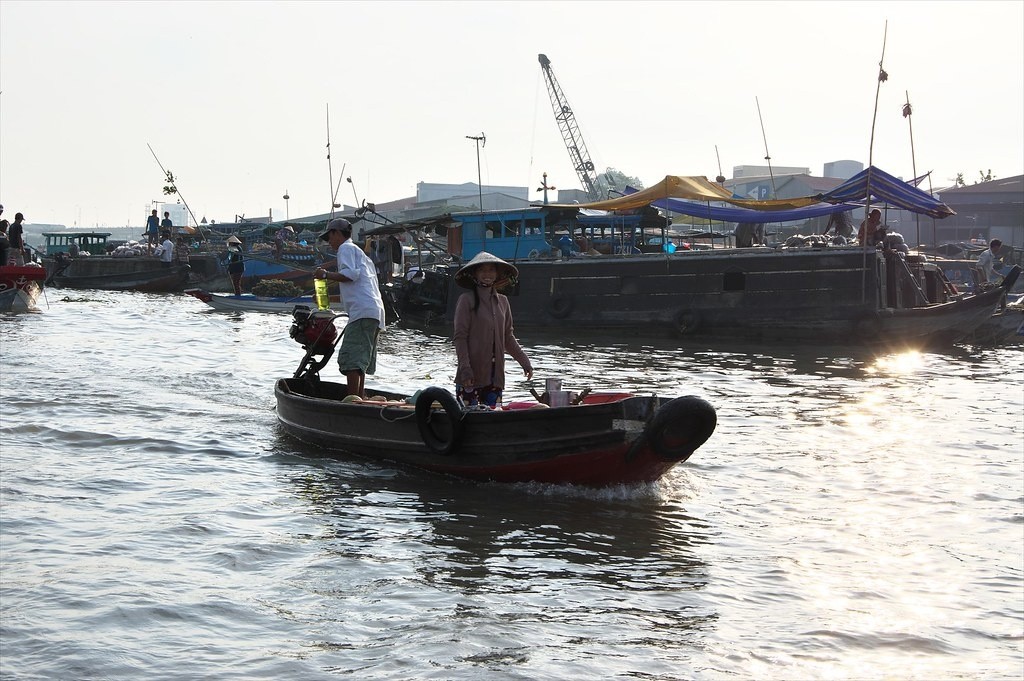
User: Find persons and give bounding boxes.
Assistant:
[823,211,853,237]
[146,210,192,282]
[452,251,532,410]
[858,209,896,255]
[732,222,766,248]
[274,225,294,261]
[975,239,1005,284]
[226,235,244,296]
[0,205,26,266]
[312,218,386,401]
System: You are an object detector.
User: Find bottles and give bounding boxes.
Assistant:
[313,277,330,310]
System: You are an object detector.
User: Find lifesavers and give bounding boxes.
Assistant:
[547,292,574,319]
[671,304,703,335]
[415,387,467,456]
[647,395,717,458]
[848,307,884,340]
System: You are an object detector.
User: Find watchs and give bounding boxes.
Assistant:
[323,270,329,280]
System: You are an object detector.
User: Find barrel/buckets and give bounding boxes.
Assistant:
[663,238,676,253]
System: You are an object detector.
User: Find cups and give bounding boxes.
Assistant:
[546,378,562,389]
[549,391,570,407]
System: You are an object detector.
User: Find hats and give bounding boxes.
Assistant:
[227,235,242,244]
[15,213,25,220]
[317,218,353,241]
[284,226,294,233]
[868,208,882,220]
[454,250,519,290]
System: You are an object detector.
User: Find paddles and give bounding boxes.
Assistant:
[222,259,238,295]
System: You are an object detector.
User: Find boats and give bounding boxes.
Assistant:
[366,171,1024,352]
[0,264,48,315]
[30,216,443,292]
[184,288,344,311]
[273,302,717,490]
